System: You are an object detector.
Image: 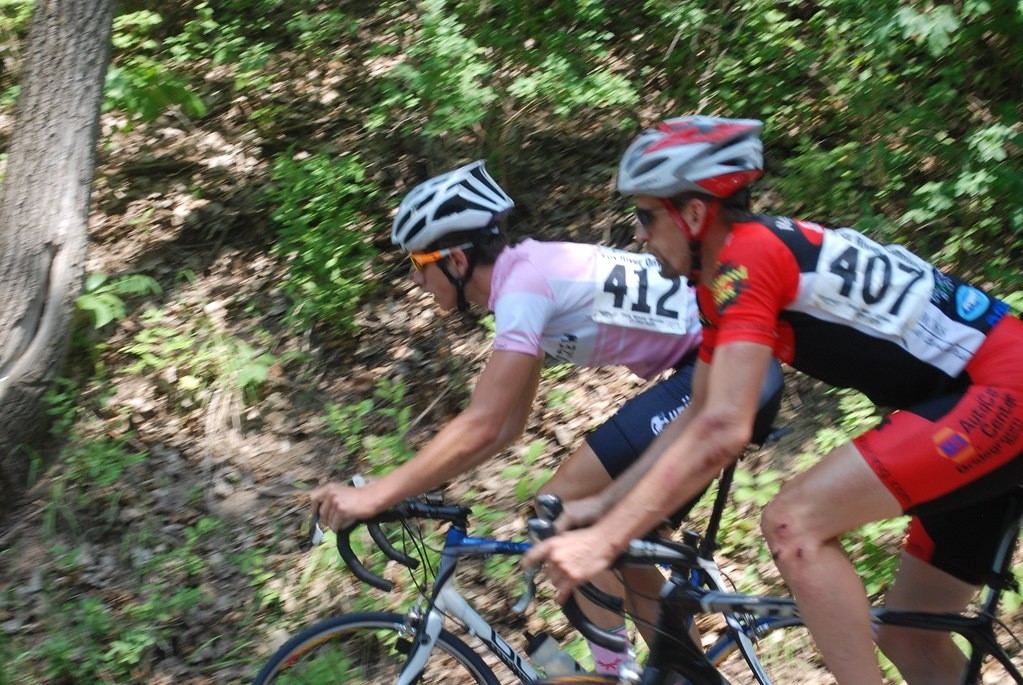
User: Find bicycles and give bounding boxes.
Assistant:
[254,425,840,684]
[505,481,1023,685]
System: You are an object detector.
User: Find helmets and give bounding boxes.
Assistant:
[617,116,762,201]
[390,159,515,253]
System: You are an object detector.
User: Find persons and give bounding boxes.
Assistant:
[519,114,1023,685]
[309,153,785,676]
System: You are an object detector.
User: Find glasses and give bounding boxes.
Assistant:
[409,242,474,271]
[633,203,668,232]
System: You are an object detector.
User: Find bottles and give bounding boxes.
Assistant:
[518,626,587,678]
